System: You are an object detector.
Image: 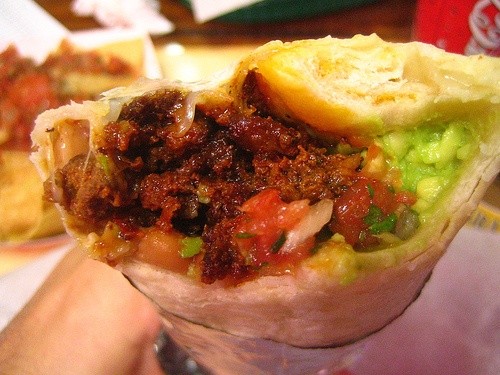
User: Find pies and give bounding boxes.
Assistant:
[21,31,500,348]
